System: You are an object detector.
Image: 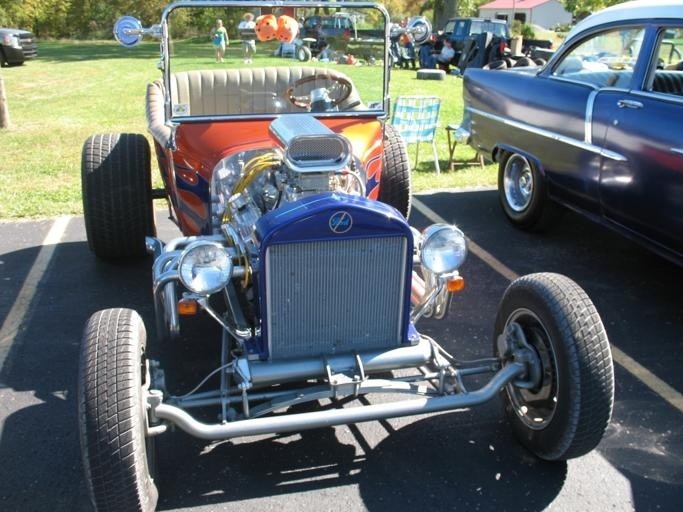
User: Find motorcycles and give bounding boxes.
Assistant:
[388,20,420,69]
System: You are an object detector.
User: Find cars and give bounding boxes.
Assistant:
[0,27,37,67]
[455,1,683,268]
[74,1,617,512]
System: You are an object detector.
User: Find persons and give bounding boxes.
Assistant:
[238,12,259,64]
[489,40,512,67]
[399,16,456,69]
[210,19,230,63]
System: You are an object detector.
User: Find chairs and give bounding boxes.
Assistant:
[446,97,484,170]
[394,97,441,174]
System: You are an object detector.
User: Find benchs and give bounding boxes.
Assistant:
[144,66,360,147]
[578,71,683,95]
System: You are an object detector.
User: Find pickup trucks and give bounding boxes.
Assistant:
[437,15,551,65]
[301,14,385,60]
